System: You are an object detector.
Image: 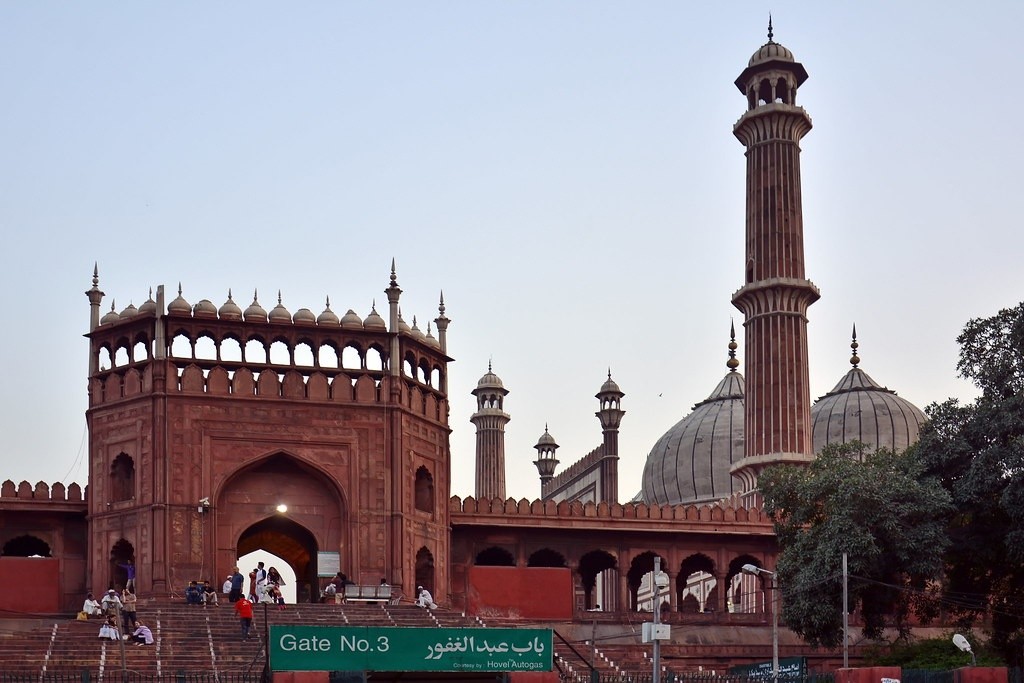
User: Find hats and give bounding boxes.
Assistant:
[418,586,423,589]
[227,575,233,579]
[108,589,115,593]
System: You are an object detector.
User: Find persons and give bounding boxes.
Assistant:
[331,571,344,603]
[117,557,137,596]
[102,613,121,641]
[267,566,280,602]
[102,589,124,616]
[223,574,232,593]
[84,591,102,618]
[416,585,434,608]
[255,561,267,603]
[185,580,202,604]
[371,578,389,604]
[588,603,604,611]
[702,607,713,613]
[131,619,154,646]
[249,568,261,603]
[199,580,219,607]
[233,593,254,641]
[228,565,244,602]
[120,586,139,638]
[338,574,351,586]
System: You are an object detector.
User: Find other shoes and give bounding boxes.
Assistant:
[202,602,207,606]
[215,603,218,607]
[132,642,144,647]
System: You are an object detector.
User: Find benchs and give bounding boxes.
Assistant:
[341,585,391,605]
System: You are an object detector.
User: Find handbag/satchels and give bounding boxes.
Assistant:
[98,624,110,638]
[76,611,90,621]
